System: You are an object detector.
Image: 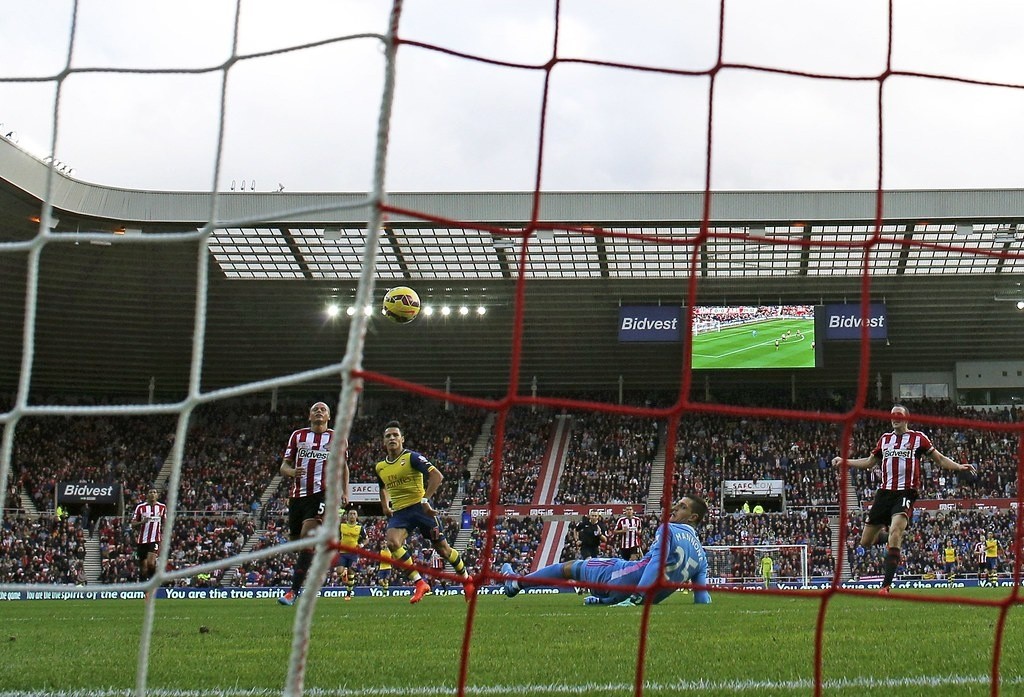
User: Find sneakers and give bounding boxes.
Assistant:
[501,563,521,597]
[344,596,351,600]
[878,587,891,595]
[409,579,430,604]
[278,590,298,606]
[463,576,475,602]
[582,596,600,606]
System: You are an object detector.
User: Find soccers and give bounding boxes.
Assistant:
[382,285,421,326]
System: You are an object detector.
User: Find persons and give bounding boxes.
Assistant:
[613,505,644,567]
[337,508,367,600]
[574,508,608,599]
[752,327,815,351]
[691,306,816,322]
[374,421,476,604]
[1,391,1023,592]
[831,403,977,595]
[131,487,167,603]
[501,493,712,605]
[374,535,399,597]
[278,402,350,605]
